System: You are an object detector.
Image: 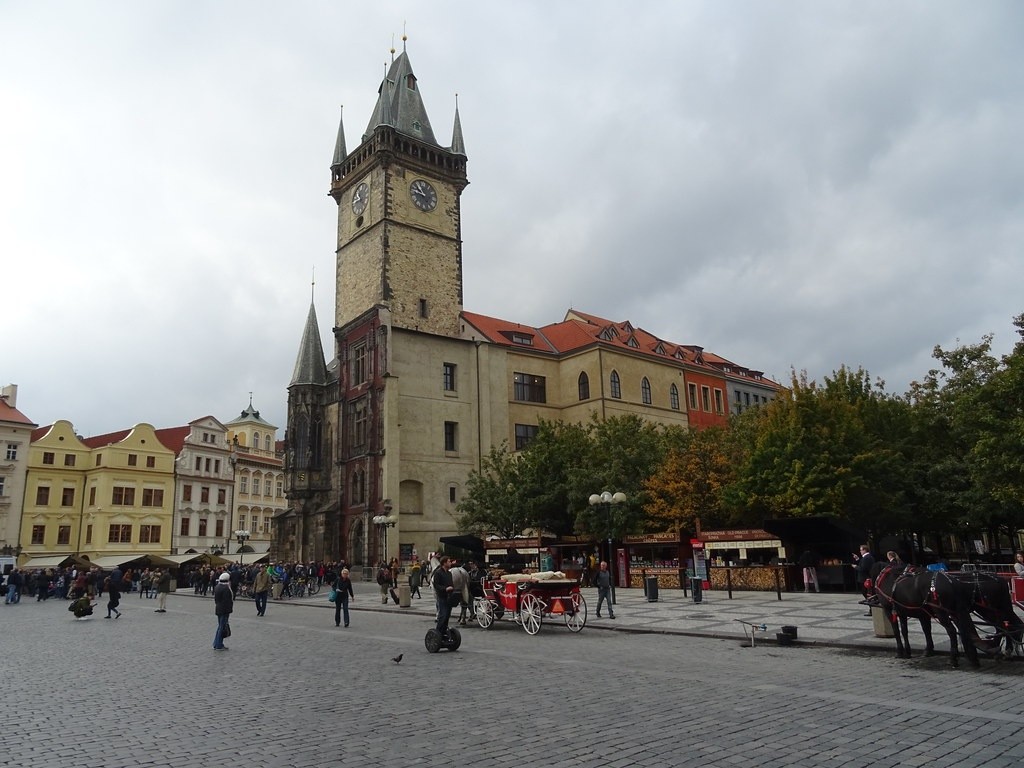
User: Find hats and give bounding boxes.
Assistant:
[259,564,265,568]
[219,573,231,582]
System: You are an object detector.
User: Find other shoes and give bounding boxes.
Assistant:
[336,624,339,626]
[115,613,121,619]
[154,609,166,612]
[345,623,349,627]
[260,612,264,617]
[213,646,229,650]
[417,597,421,599]
[864,613,872,616]
[76,617,87,621]
[596,612,601,618]
[257,608,262,615]
[610,615,615,619]
[104,615,111,618]
[410,596,413,599]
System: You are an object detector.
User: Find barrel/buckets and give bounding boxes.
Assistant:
[781,625,798,638]
[776,632,792,647]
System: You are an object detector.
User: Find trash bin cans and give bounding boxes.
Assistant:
[690,576,703,604]
[646,576,660,603]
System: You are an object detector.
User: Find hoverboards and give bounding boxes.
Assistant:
[425,589,462,654]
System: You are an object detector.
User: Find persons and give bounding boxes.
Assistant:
[377,556,399,604]
[1013,551,1024,578]
[432,555,454,642]
[0,565,161,604]
[411,569,421,600]
[186,560,350,598]
[850,545,875,616]
[154,570,170,613]
[253,565,271,618]
[415,559,431,587]
[886,551,903,565]
[593,561,615,619]
[73,593,97,620]
[332,569,354,626]
[796,546,821,593]
[103,577,122,620]
[582,551,592,588]
[212,572,234,650]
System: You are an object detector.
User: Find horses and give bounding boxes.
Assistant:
[427,551,477,626]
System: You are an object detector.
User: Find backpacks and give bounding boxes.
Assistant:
[68,597,88,611]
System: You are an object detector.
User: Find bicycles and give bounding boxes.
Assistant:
[236,570,322,599]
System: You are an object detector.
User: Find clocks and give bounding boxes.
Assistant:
[352,183,370,216]
[409,180,438,211]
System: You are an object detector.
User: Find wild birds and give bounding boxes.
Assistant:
[390,653,404,664]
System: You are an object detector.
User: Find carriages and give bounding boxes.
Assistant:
[425,552,588,636]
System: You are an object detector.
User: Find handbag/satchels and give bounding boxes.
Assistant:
[223,621,231,638]
[329,577,340,602]
[447,588,461,607]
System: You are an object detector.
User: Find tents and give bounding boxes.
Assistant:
[23,552,271,568]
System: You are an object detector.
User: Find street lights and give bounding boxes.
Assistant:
[235,529,250,566]
[372,515,399,565]
[589,492,629,605]
